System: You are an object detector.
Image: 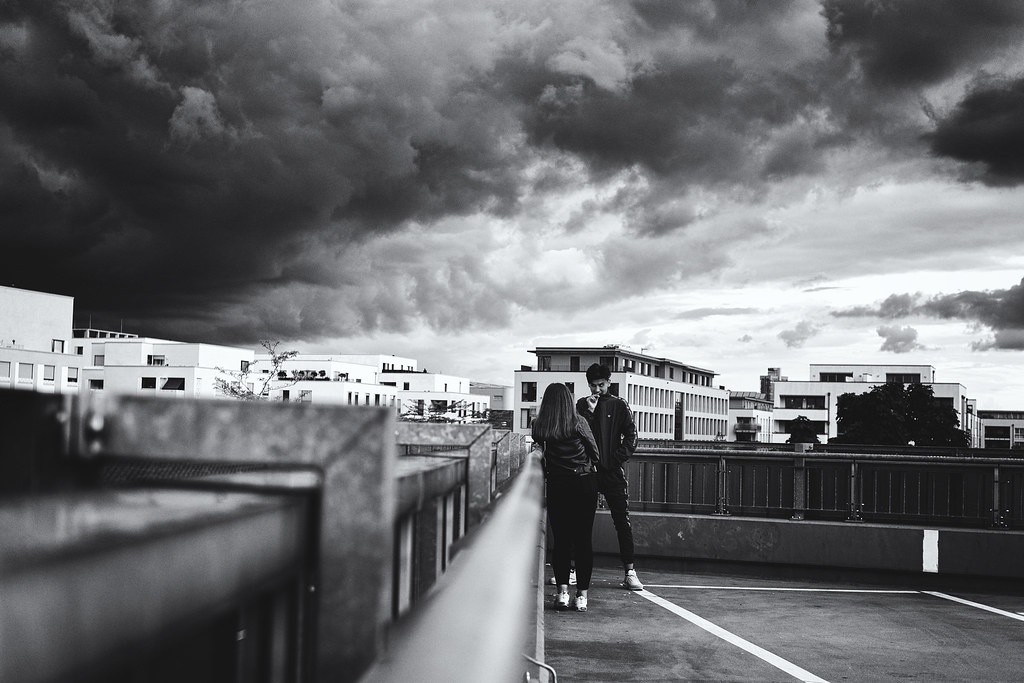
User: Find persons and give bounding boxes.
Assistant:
[548,364,646,591]
[532,383,600,613]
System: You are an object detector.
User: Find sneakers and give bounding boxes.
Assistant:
[554,591,570,609]
[549,569,577,585]
[624,570,643,591]
[572,595,587,611]
